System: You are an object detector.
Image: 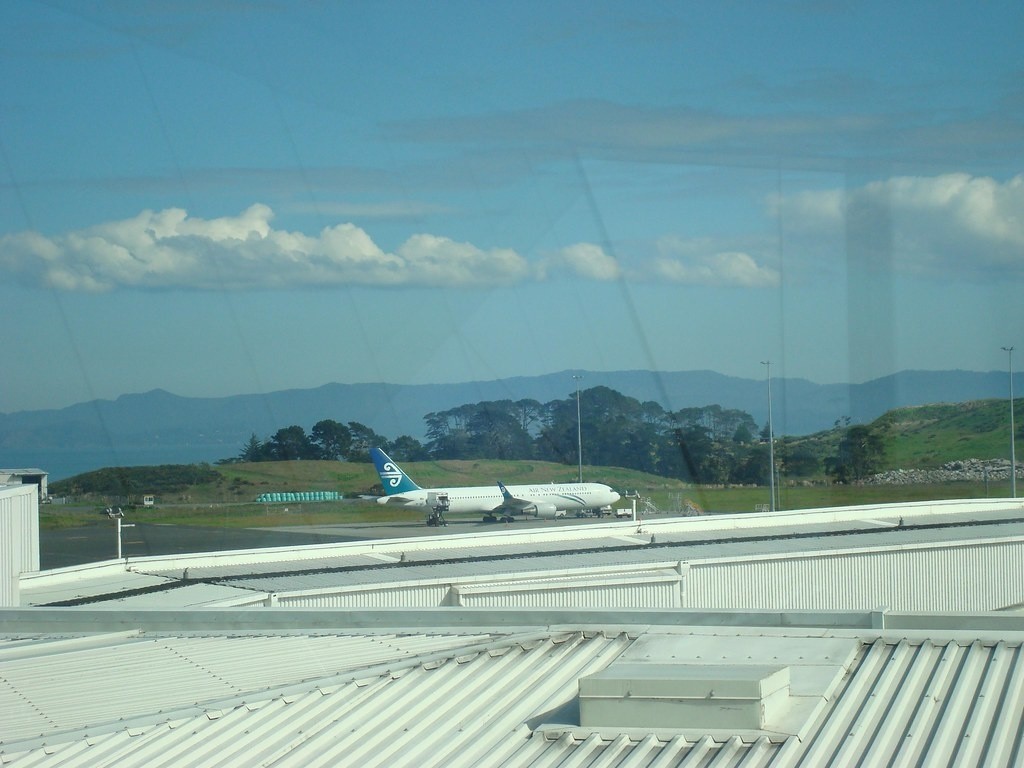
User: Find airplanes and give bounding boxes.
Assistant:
[357,445,622,524]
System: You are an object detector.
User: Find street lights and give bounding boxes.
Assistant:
[572,375,582,482]
[1000,344,1017,499]
[759,361,777,512]
[105,507,125,559]
